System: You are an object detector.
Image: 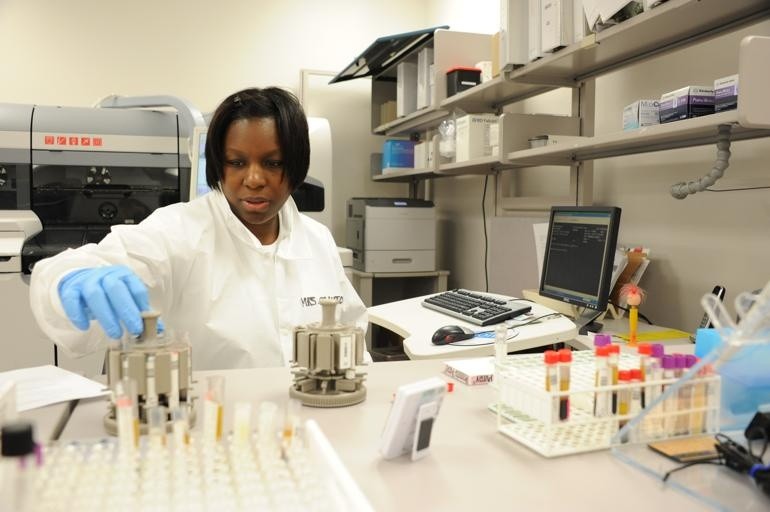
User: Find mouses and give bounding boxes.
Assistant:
[432,325,475,345]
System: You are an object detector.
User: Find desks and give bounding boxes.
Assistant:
[67,337,769,512]
[566,321,679,349]
[4,375,70,444]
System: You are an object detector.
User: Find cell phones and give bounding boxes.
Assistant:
[700,285,725,328]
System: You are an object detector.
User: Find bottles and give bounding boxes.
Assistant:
[544,333,713,443]
[494,324,507,385]
[115,368,306,466]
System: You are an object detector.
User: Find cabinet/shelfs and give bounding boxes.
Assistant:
[365,0,770,182]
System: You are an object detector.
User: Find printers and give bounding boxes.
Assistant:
[346,197,436,273]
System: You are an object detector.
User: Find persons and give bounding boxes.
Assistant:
[30,88,373,371]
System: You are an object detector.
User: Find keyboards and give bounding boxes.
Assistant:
[422,288,531,326]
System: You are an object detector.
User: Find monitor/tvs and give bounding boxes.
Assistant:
[539,206,621,335]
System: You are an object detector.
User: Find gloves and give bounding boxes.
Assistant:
[57,267,164,338]
